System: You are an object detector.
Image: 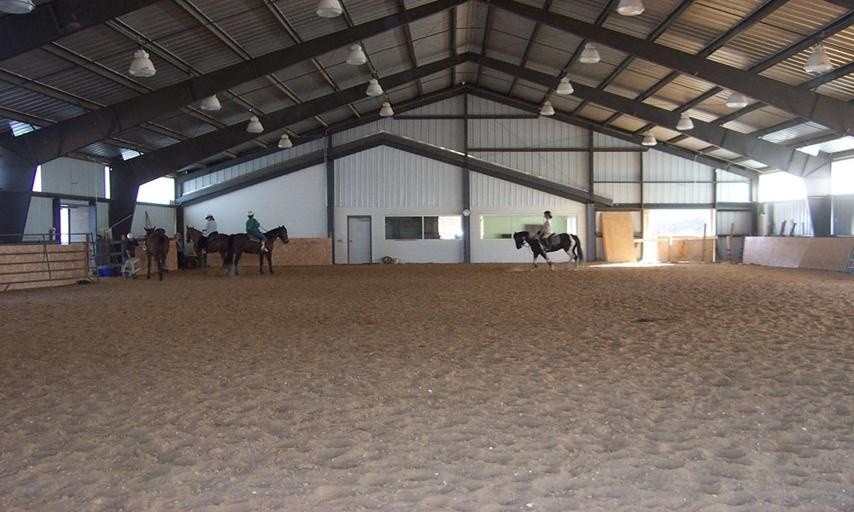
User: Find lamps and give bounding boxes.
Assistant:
[346,44,367,65]
[316,1,342,18]
[380,99,392,117]
[617,1,644,16]
[803,40,833,72]
[725,93,748,108]
[556,77,573,94]
[0,1,36,16]
[366,71,383,96]
[278,134,293,148]
[642,130,657,145]
[676,113,693,130]
[200,94,221,110]
[129,45,156,78]
[540,100,555,115]
[246,116,264,133]
[579,41,600,63]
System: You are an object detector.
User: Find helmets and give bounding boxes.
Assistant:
[204,214,212,219]
[247,211,253,216]
[126,233,133,239]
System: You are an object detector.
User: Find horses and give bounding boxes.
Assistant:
[223,225,289,278]
[143,225,169,282]
[513,231,585,272]
[185,224,231,269]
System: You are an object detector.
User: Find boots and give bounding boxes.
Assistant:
[543,238,551,251]
[260,240,268,252]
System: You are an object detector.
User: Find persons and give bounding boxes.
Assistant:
[121,233,139,280]
[199,215,218,255]
[246,210,269,252]
[536,211,553,252]
